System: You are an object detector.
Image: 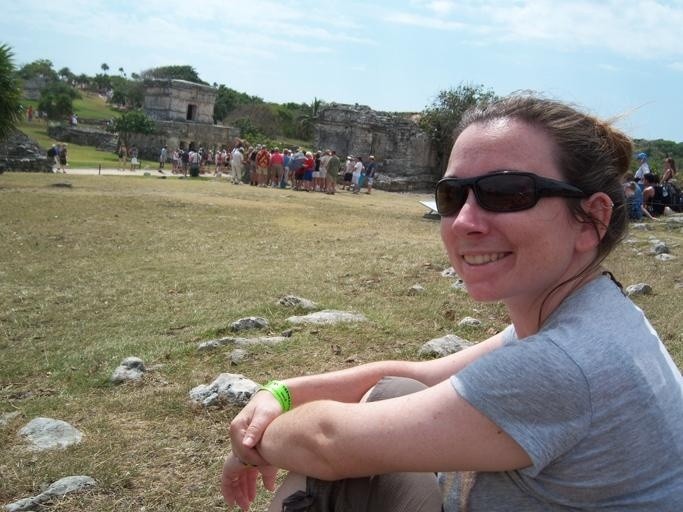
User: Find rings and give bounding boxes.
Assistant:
[231,476,239,482]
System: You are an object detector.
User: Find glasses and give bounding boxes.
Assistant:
[434,169,582,218]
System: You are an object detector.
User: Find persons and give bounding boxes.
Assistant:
[221,99,682,512]
[26,104,32,120]
[128,144,139,170]
[158,141,377,195]
[119,143,128,168]
[47,143,68,173]
[623,153,683,220]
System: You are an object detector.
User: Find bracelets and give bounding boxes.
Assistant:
[237,460,258,469]
[254,378,292,412]
